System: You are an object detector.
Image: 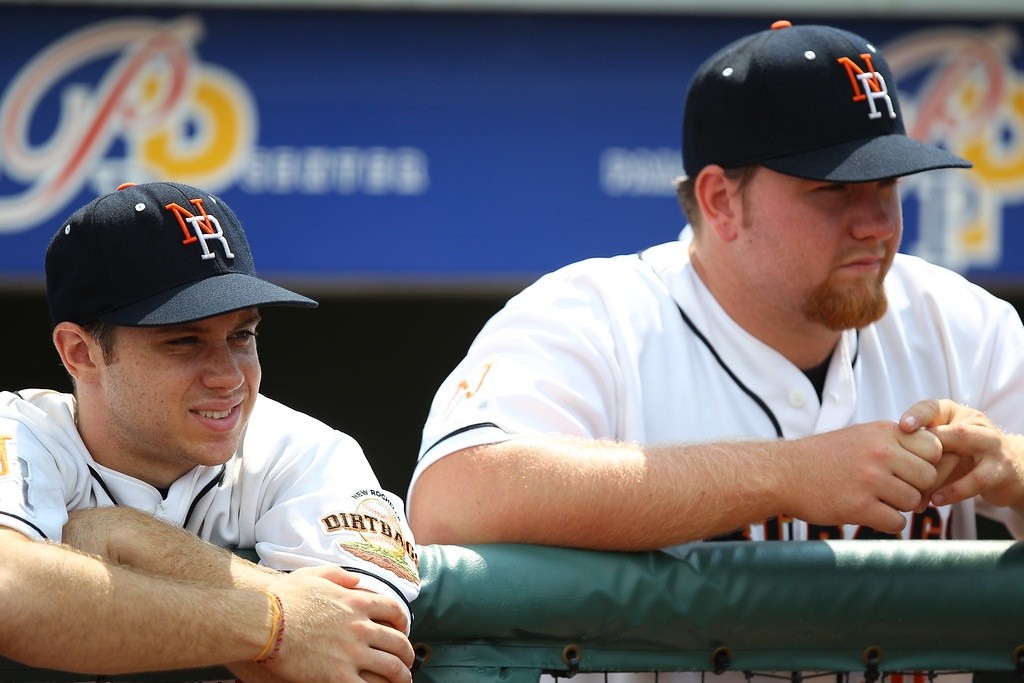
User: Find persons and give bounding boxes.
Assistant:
[405,18,1023,683]
[0,179,420,681]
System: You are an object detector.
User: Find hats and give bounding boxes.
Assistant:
[684,20,974,182]
[45,183,318,327]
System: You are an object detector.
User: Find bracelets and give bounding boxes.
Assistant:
[253,588,285,667]
